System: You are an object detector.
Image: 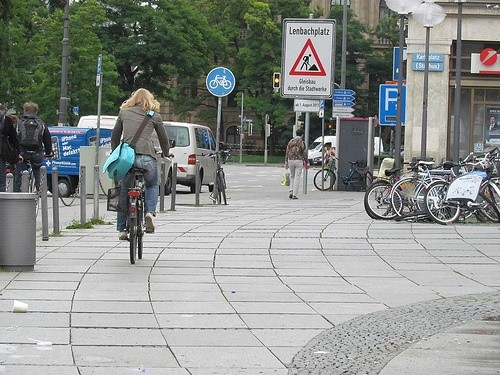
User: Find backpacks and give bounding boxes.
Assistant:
[17,117,44,152]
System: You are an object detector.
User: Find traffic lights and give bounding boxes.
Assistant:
[273,72,281,88]
[328,123,332,129]
[300,121,304,129]
[271,125,273,135]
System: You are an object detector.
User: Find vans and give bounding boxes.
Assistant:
[39,125,115,199]
[157,121,225,193]
[78,114,119,129]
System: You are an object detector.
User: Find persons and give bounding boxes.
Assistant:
[488,115,500,131]
[111,88,173,241]
[324,146,335,166]
[17,102,52,198]
[285,129,310,199]
[0,105,23,191]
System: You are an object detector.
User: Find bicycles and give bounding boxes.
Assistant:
[18,149,54,217]
[110,151,173,264]
[364,147,500,225]
[313,153,374,192]
[207,149,231,205]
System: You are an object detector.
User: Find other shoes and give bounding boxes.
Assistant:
[119,230,129,240]
[288,190,293,198]
[144,212,156,234]
[292,196,298,199]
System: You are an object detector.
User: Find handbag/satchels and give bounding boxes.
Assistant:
[0,110,21,164]
[280,167,290,186]
[102,142,136,182]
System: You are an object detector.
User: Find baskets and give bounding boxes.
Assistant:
[356,159,367,167]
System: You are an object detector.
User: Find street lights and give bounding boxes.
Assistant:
[385,0,447,218]
